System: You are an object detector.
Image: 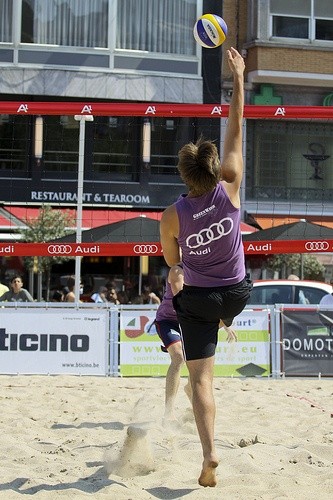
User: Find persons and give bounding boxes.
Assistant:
[154,247,238,422]
[159,46,245,487]
[0,274,163,304]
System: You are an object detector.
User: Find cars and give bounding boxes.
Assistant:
[239,279,333,305]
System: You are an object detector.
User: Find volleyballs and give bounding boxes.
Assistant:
[193,13,228,49]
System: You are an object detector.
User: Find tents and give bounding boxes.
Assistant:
[0,216,333,256]
